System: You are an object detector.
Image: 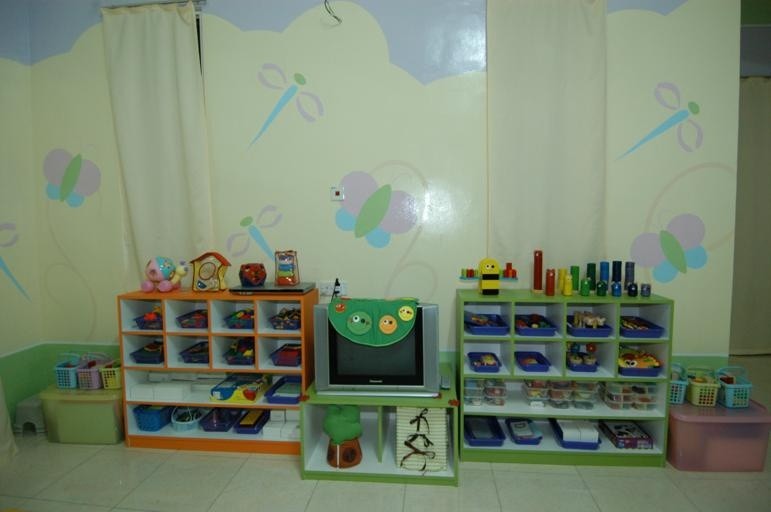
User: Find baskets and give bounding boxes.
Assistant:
[719,365,754,409]
[669,363,690,405]
[464,309,666,377]
[263,374,302,404]
[133,406,265,434]
[464,415,602,450]
[130,308,301,366]
[687,367,723,407]
[77,352,113,390]
[394,406,448,473]
[53,352,80,390]
[99,359,123,391]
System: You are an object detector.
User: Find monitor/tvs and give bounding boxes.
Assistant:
[312,303,440,398]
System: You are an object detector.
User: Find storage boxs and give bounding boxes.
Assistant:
[38,381,126,446]
[666,402,771,473]
[131,404,173,432]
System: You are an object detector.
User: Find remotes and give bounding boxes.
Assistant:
[440,374,450,390]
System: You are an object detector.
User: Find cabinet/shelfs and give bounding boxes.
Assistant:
[297,362,469,493]
[114,287,322,456]
[449,285,678,467]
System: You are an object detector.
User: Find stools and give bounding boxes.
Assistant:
[10,394,48,433]
